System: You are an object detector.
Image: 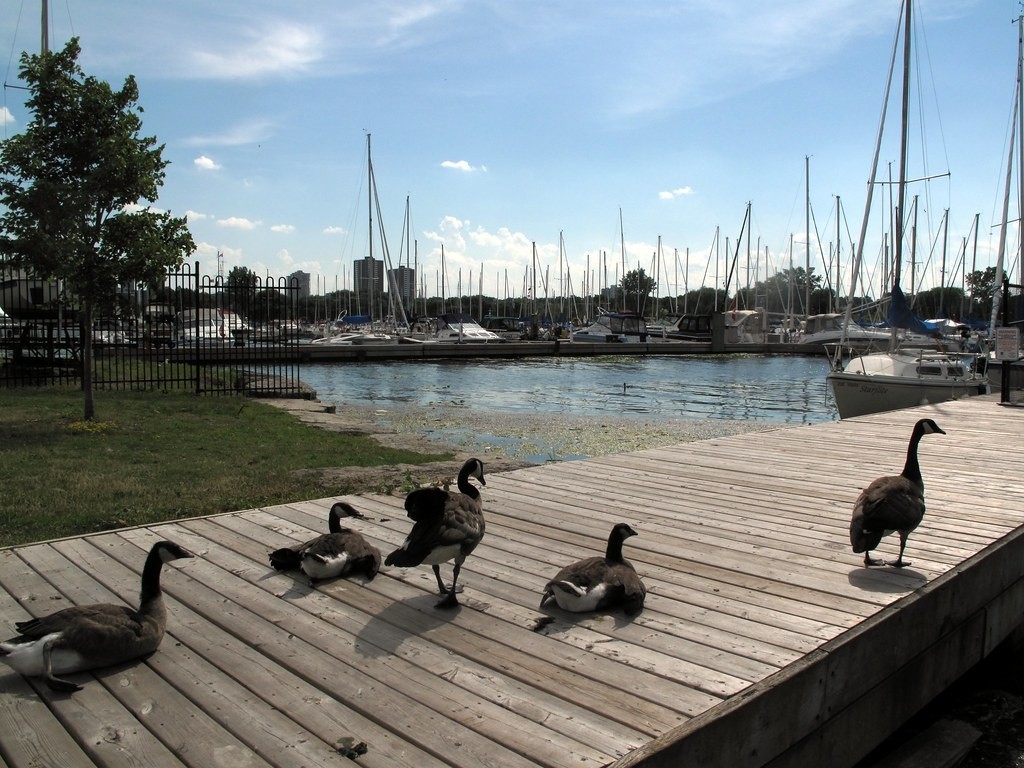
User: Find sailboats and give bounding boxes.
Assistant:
[823,6,990,421]
[94,130,1023,344]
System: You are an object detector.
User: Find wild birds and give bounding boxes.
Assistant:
[539,522,647,615]
[0,541,196,694]
[267,502,382,589]
[383,457,486,610]
[848,418,947,567]
